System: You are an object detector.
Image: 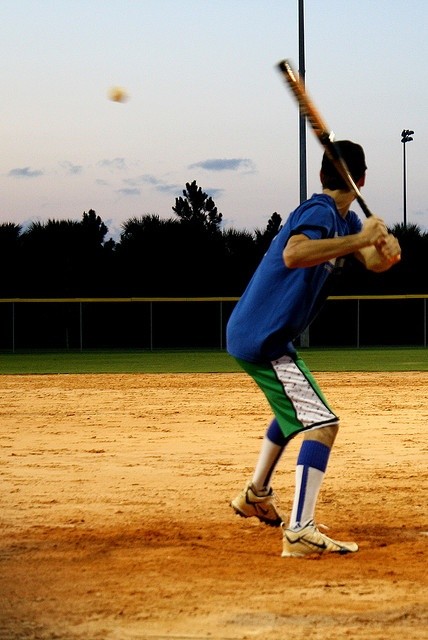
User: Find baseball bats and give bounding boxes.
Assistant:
[279,58,402,264]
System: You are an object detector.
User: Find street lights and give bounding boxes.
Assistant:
[402,128,413,229]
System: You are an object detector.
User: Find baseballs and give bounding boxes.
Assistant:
[109,86,126,102]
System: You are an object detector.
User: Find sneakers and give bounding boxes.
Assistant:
[280,513,359,558]
[232,481,284,528]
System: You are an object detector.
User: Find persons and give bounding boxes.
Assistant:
[226,139,403,557]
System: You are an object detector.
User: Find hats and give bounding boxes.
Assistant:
[322,141,367,178]
